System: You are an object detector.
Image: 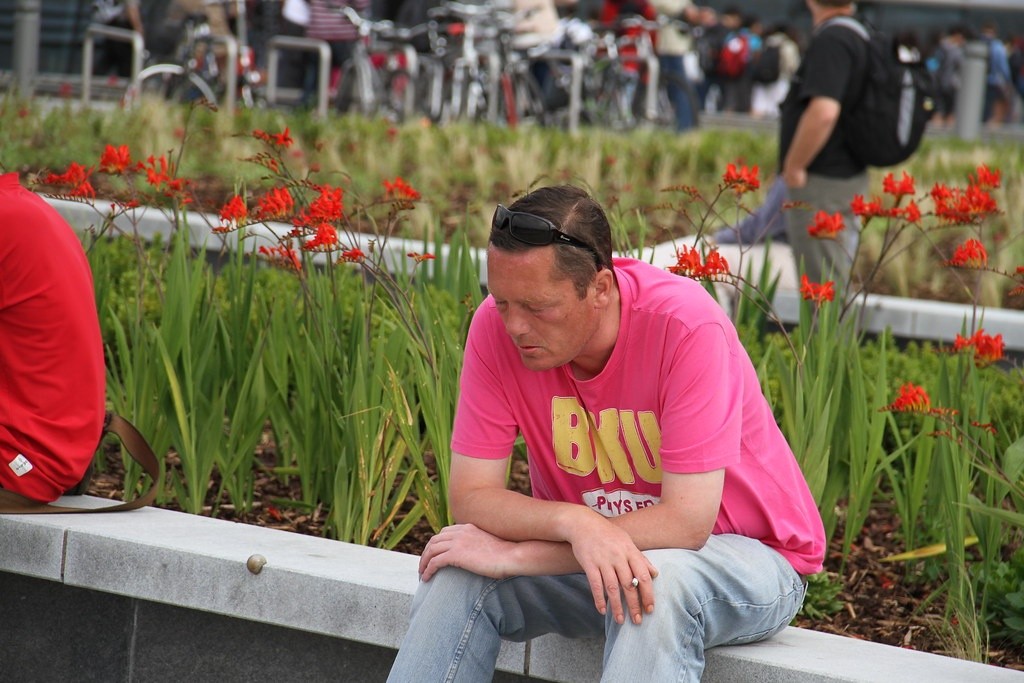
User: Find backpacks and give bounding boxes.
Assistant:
[749,35,790,85]
[806,12,937,166]
[715,28,749,80]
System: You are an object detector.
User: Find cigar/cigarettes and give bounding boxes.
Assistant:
[632,577,639,588]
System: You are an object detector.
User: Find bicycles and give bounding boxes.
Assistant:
[106,0,724,131]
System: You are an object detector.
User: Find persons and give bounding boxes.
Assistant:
[383,185,831,682]
[709,0,881,340]
[87,1,1023,128]
[0,168,109,509]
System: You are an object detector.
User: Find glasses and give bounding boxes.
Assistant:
[492,204,603,272]
